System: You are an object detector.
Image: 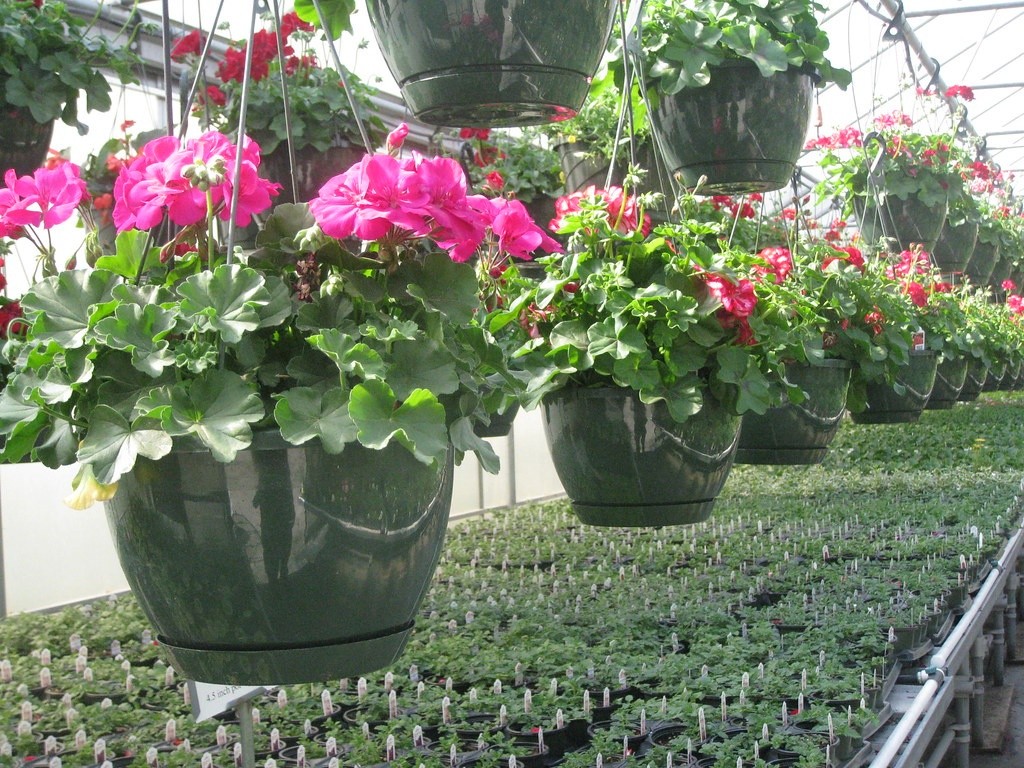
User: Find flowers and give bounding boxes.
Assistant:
[0,12,1024,353]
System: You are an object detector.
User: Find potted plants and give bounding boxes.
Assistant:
[0,1,159,189]
[539,70,680,228]
[1,391,1024,767]
[607,0,851,196]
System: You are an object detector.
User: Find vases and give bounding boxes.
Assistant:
[736,359,850,466]
[504,201,562,264]
[964,240,998,284]
[257,142,368,219]
[929,222,978,273]
[981,362,1008,392]
[957,360,989,402]
[998,363,1021,391]
[537,387,742,526]
[367,0,618,129]
[1010,272,1022,297]
[1013,365,1024,391]
[923,357,969,409]
[853,194,947,255]
[93,431,456,685]
[987,254,1012,294]
[846,353,936,424]
